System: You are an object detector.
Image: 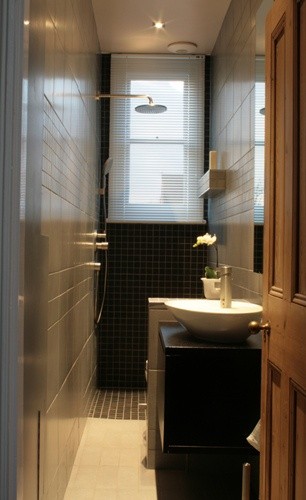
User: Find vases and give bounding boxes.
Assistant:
[201,279,222,299]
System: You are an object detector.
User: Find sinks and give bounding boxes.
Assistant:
[164,299,263,341]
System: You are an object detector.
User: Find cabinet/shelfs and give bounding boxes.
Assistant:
[157,321,261,456]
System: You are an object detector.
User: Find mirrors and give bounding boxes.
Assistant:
[254,0,274,274]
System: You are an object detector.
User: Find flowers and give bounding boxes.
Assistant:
[192,233,219,278]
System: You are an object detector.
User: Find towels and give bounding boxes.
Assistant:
[246,417,261,452]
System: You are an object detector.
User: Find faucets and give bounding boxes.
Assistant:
[215,265,233,308]
